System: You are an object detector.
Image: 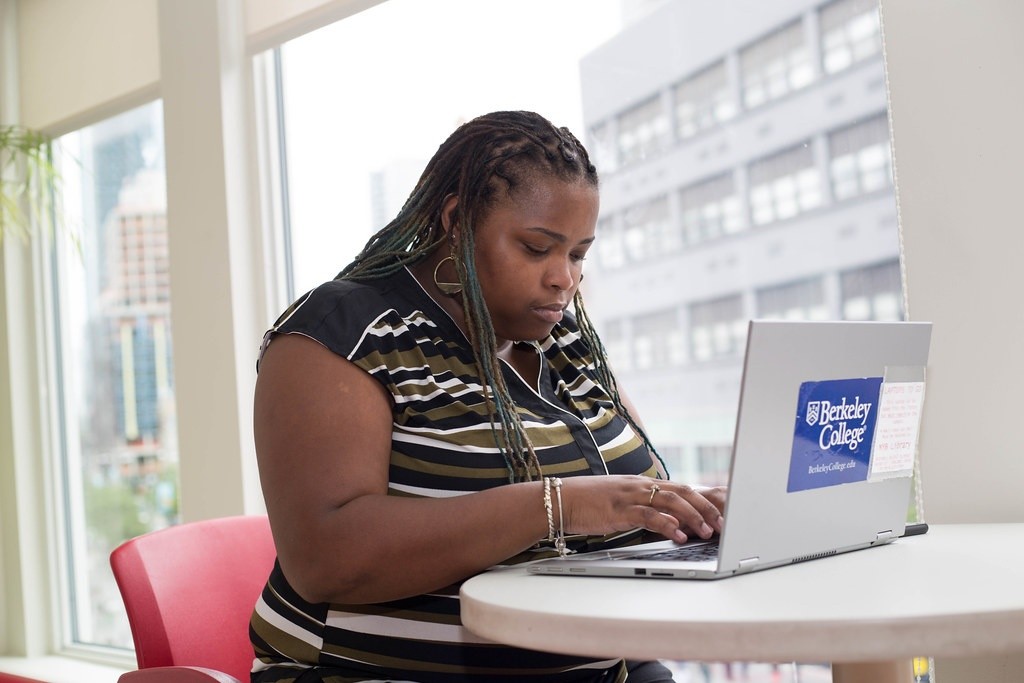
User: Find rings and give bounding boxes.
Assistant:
[649,484,660,504]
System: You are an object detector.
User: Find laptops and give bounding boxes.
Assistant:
[528,321,933,578]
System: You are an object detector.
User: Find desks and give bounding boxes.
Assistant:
[460,522,1024,683]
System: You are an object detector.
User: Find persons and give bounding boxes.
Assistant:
[249,111,727,683]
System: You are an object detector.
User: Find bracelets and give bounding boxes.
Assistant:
[543,476,567,552]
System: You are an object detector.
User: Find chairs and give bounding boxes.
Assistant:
[109,513,276,683]
